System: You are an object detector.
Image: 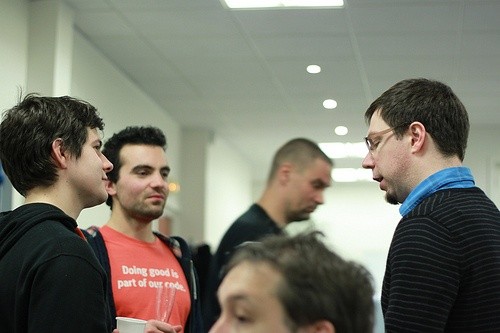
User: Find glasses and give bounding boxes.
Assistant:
[364,121,412,150]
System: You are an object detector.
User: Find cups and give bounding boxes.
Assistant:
[115,317,147,333]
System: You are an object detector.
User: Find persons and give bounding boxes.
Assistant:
[208,230,377,333]
[0,95,114,333]
[205,138,334,333]
[362,78,500,333]
[80,126,204,333]
